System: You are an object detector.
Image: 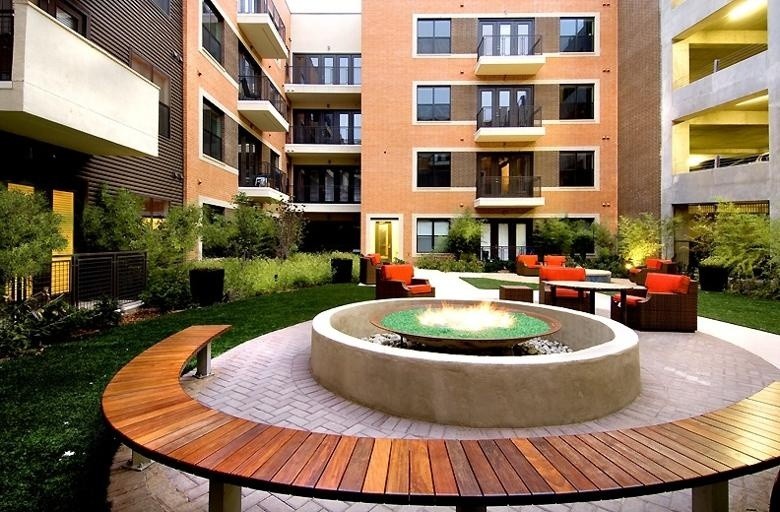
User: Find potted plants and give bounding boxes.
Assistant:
[696,253,742,290]
[189,262,223,306]
[332,252,353,283]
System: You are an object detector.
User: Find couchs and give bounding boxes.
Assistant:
[516,254,702,331]
[360,253,435,299]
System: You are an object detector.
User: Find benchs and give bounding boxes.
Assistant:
[102,324,780,512]
